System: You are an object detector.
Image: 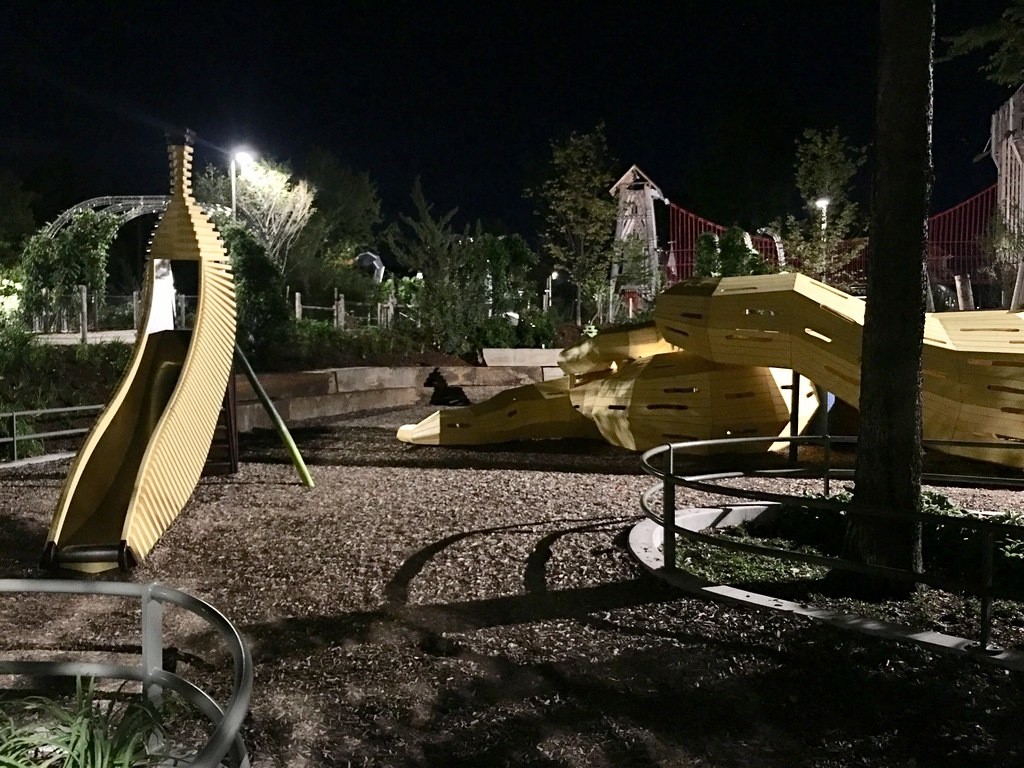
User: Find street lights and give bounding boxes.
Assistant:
[229,151,255,224]
[814,199,830,285]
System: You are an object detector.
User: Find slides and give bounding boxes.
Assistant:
[35,331,236,573]
